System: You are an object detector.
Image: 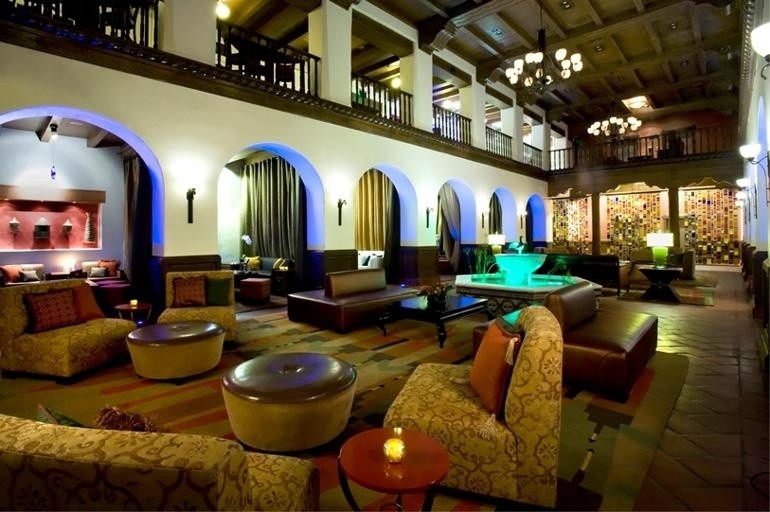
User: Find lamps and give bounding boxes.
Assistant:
[646,232,674,268]
[487,231,506,255]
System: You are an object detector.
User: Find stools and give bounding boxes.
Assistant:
[240,278,272,306]
[126,320,357,453]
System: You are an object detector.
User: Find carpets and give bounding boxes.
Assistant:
[618,286,715,306]
[0,311,689,512]
[630,271,719,287]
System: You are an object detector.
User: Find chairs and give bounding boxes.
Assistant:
[0,271,235,385]
[384,304,563,507]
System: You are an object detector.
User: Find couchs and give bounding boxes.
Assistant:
[230,256,295,296]
[472,281,658,387]
[0,406,320,512]
[287,268,417,336]
[533,247,696,296]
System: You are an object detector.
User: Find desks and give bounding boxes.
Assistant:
[379,296,489,348]
[639,266,682,303]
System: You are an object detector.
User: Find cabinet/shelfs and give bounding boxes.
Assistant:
[0,184,106,252]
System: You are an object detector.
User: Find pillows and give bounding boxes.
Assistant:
[0,259,230,331]
[38,404,88,428]
[469,315,521,411]
[244,256,288,271]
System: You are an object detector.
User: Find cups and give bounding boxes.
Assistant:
[384,424,406,463]
[130,299,138,309]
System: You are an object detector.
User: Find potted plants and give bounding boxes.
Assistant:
[418,279,454,308]
